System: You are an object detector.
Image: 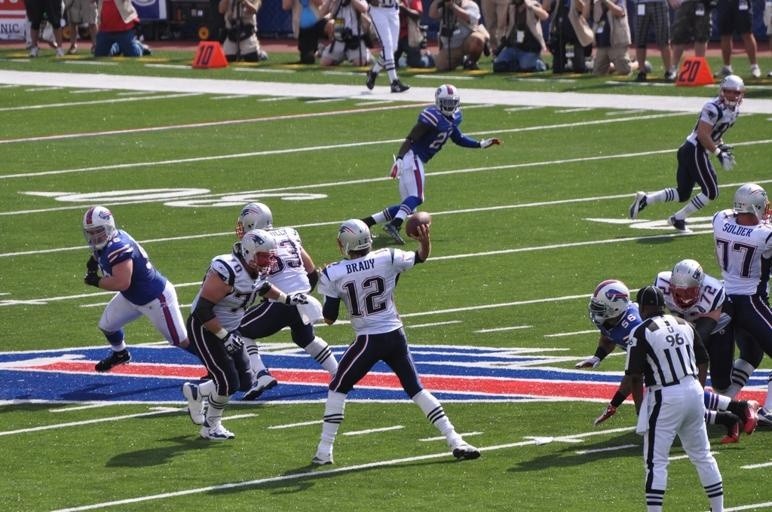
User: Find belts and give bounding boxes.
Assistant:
[367,2,396,9]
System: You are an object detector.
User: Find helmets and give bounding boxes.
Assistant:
[83,205,116,253]
[733,183,768,225]
[671,259,705,312]
[718,75,744,107]
[585,278,629,331]
[236,202,372,271]
[435,84,460,116]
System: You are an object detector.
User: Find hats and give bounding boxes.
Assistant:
[636,285,664,306]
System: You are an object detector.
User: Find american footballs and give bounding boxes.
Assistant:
[407,212,432,237]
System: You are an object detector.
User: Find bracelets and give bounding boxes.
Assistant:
[276,290,289,306]
[594,345,609,361]
[609,390,625,409]
[214,328,228,340]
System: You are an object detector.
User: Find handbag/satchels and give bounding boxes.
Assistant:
[334,28,360,50]
[228,24,253,41]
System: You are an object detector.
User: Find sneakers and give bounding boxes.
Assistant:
[464,60,478,70]
[451,442,480,459]
[383,223,405,245]
[367,70,377,89]
[180,368,333,466]
[749,63,761,79]
[391,78,409,92]
[712,67,731,78]
[720,400,772,443]
[667,216,691,235]
[665,68,678,83]
[626,191,646,219]
[94,351,131,372]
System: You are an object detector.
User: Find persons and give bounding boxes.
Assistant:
[361,84,500,245]
[628,74,744,233]
[218,0,269,62]
[182,228,309,441]
[236,201,340,400]
[575,279,759,441]
[712,184,772,425]
[82,205,202,372]
[24,0,151,57]
[308,219,483,469]
[654,259,736,398]
[593,284,728,511]
[281,3,763,91]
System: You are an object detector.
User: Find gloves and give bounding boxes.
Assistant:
[594,403,616,424]
[84,256,101,287]
[574,356,601,368]
[286,292,309,305]
[390,158,402,180]
[714,143,736,170]
[223,332,244,356]
[480,137,501,149]
